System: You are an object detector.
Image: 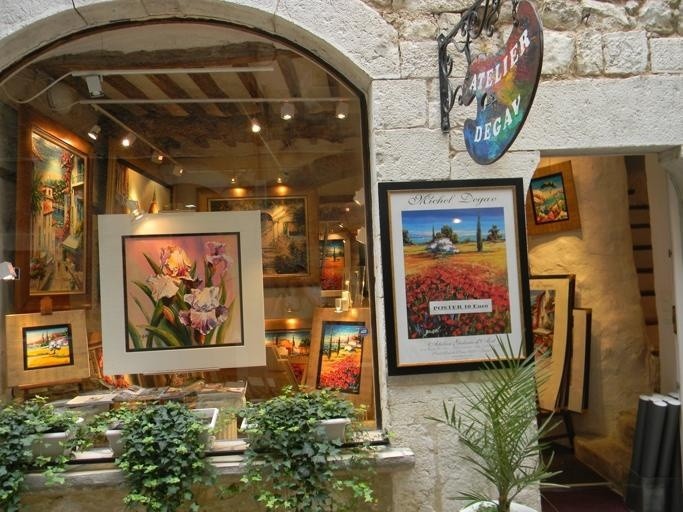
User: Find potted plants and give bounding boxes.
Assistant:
[93,399,230,512]
[0,395,96,512]
[422,325,577,512]
[228,382,387,512]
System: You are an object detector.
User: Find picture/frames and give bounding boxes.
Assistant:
[193,182,324,287]
[94,209,269,380]
[299,305,373,417]
[523,161,585,236]
[314,227,354,301]
[101,133,179,214]
[13,105,94,315]
[5,306,93,392]
[377,177,536,375]
